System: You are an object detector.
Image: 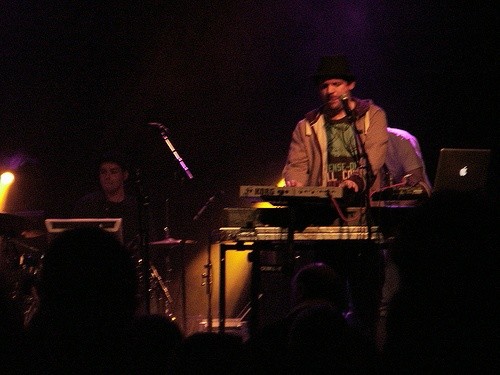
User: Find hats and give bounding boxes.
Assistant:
[311,62,360,92]
[97,152,128,171]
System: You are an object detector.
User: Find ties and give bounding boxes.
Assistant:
[381,163,389,189]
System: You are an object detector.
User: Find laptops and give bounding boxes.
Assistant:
[429,148,491,194]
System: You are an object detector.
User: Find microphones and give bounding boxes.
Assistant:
[340,95,350,110]
[193,196,214,220]
[149,122,170,133]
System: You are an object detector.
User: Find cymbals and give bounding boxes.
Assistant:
[147,239,199,248]
[0,213,46,239]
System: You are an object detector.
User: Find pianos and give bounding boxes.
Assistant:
[216,183,392,329]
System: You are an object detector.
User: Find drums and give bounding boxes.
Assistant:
[130,269,165,300]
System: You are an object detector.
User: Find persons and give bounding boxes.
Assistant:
[279,54,387,226]
[384,196,500,375]
[369,127,431,194]
[69,158,143,251]
[0,229,378,375]
